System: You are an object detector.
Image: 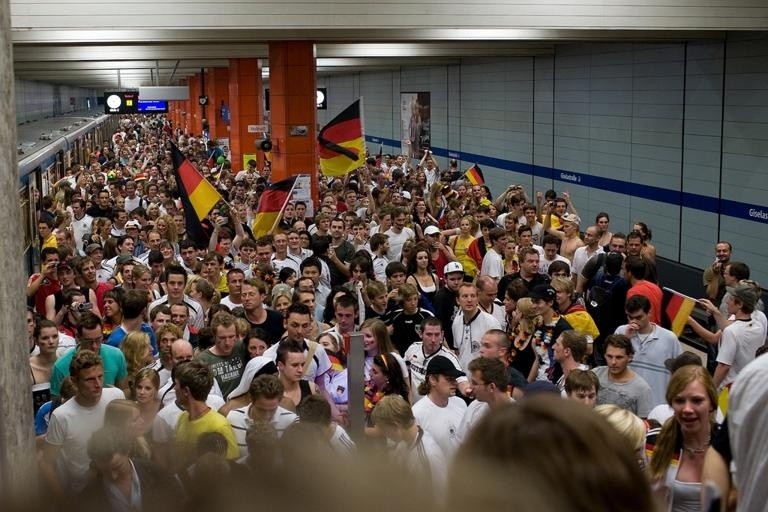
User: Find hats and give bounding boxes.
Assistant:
[56,260,71,271]
[443,262,465,274]
[116,253,133,264]
[59,179,72,186]
[400,191,412,200]
[423,225,442,236]
[605,251,623,271]
[124,219,141,233]
[85,244,101,255]
[528,284,555,298]
[561,214,580,226]
[665,351,700,373]
[425,356,466,376]
[724,285,756,306]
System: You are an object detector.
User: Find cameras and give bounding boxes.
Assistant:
[78,301,94,313]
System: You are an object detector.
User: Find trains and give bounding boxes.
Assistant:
[19,106,122,263]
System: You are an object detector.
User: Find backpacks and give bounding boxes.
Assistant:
[585,273,623,321]
